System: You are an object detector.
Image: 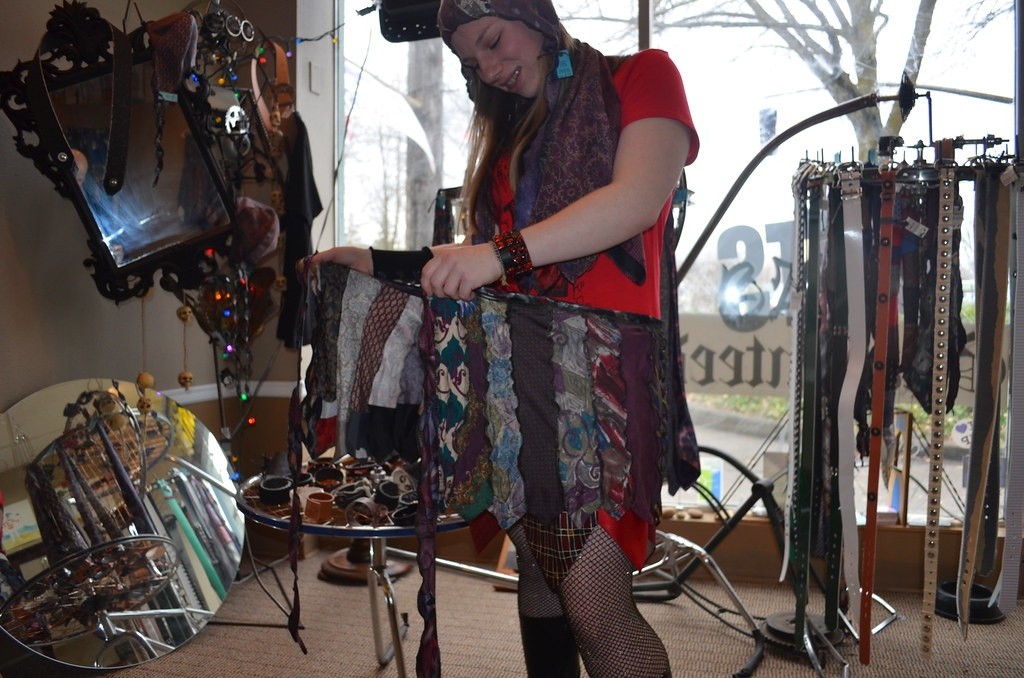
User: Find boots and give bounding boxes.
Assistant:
[517,612,581,678]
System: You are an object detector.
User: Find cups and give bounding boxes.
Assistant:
[260,446,419,526]
[3,544,165,643]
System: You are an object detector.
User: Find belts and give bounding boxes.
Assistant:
[248,38,296,180]
[102,467,243,663]
[777,138,1023,666]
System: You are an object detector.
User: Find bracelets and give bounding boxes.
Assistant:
[12,540,169,641]
[258,454,455,524]
[493,229,534,279]
[487,240,509,286]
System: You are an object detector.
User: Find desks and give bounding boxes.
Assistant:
[0,534,218,669]
[236,461,470,667]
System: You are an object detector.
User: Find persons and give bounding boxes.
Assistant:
[292,0,703,677]
[71,149,126,261]
